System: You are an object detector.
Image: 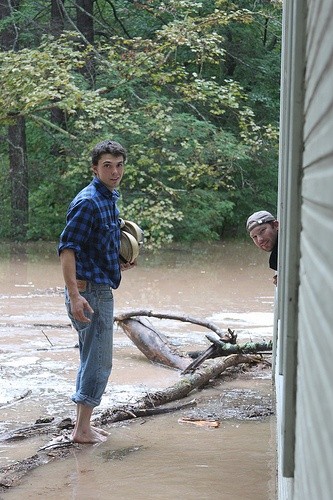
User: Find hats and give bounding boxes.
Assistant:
[246,211,275,232]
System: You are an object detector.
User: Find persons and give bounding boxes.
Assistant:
[247,210,279,286]
[56,141,137,445]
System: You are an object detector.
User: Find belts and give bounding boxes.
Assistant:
[86,281,111,292]
